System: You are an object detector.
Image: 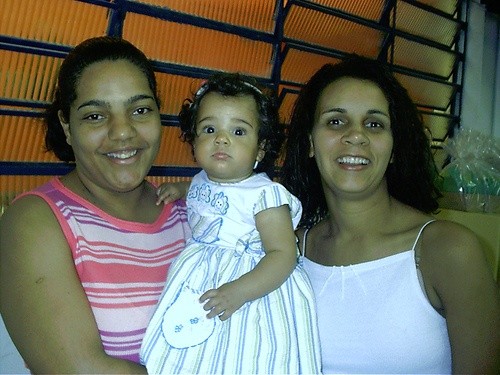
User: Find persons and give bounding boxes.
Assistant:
[0,36,190,375]
[140,71,321,375]
[276,55,500,374]
